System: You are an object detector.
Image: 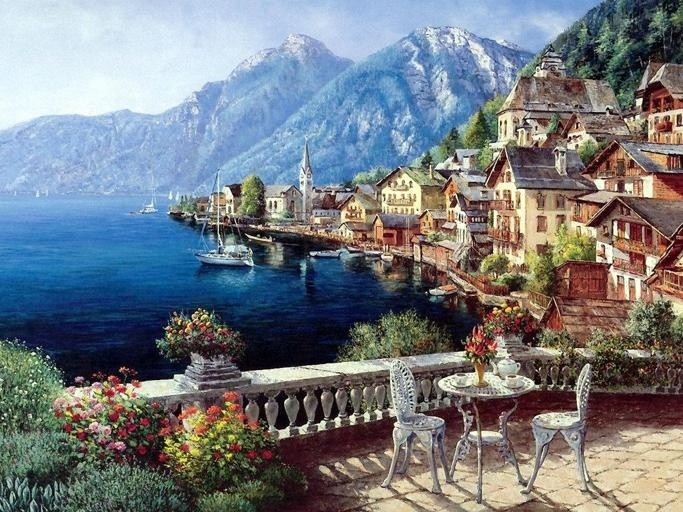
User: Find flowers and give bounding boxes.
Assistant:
[461,298,541,364]
[155,307,246,364]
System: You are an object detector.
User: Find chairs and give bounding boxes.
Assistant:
[382,357,452,495]
[521,362,592,493]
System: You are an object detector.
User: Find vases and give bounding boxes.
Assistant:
[471,361,486,387]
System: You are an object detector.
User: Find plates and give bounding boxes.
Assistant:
[449,378,473,388]
[502,379,525,388]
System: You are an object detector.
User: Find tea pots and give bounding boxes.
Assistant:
[489,354,522,380]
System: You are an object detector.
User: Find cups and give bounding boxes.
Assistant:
[504,373,521,388]
[454,373,469,384]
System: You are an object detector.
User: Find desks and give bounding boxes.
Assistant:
[435,372,533,504]
